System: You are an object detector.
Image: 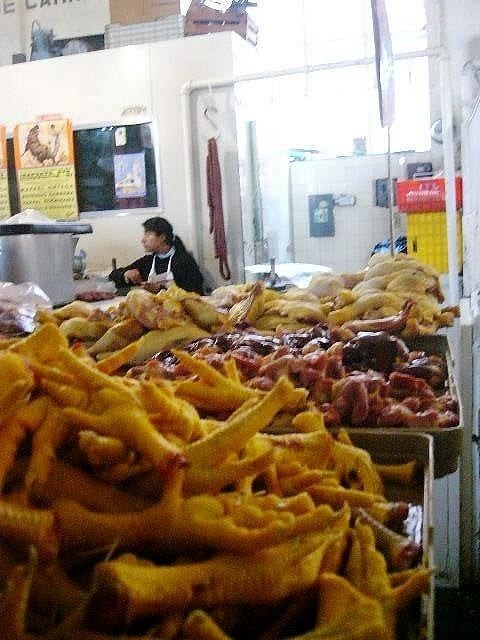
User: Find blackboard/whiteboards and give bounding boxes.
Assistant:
[5,117,164,220]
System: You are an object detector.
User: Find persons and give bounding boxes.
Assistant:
[109,217,204,294]
